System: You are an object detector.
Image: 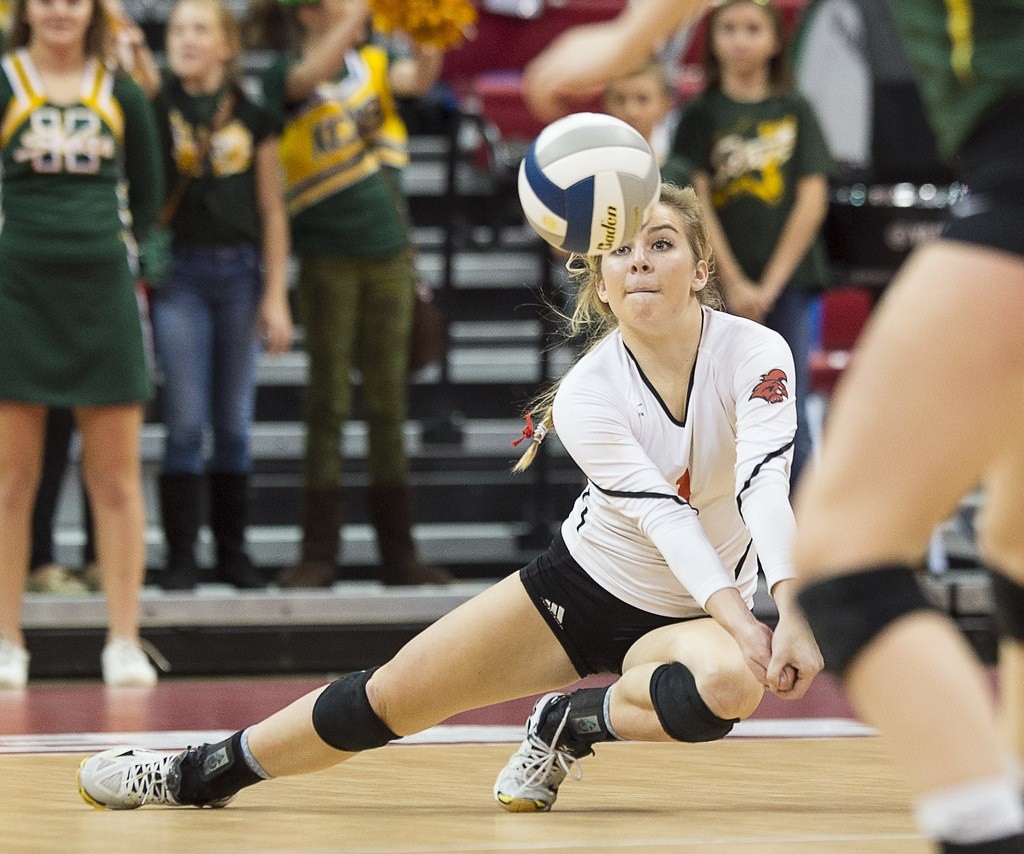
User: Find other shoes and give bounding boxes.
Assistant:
[160,552,196,588]
[0,641,30,689]
[28,561,108,593]
[277,557,341,588]
[99,637,170,688]
[219,553,271,588]
[380,557,450,584]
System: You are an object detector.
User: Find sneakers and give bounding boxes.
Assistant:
[76,743,237,811]
[494,692,595,813]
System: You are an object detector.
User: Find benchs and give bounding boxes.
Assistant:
[20,51,997,629]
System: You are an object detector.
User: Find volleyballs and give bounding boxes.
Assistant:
[517,112,662,257]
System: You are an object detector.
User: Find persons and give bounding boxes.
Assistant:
[78,182,825,814]
[789,75,1024,854]
[0,0,1024,688]
